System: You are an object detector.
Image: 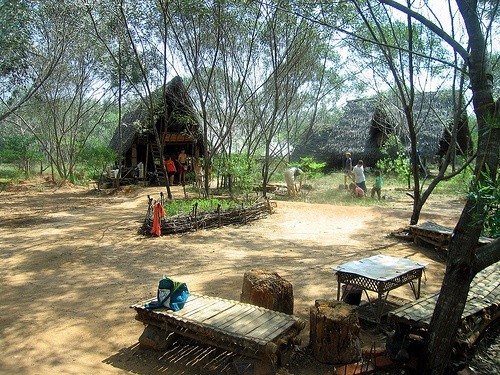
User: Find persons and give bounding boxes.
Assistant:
[178,149,188,185]
[345,153,382,201]
[285,167,307,199]
[164,157,177,186]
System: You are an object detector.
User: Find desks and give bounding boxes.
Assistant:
[331,254,431,304]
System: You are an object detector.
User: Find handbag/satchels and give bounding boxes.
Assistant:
[142,279,189,312]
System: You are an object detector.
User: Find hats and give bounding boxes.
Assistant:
[359,160,363,164]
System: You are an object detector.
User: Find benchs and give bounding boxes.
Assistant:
[132,286,307,375]
